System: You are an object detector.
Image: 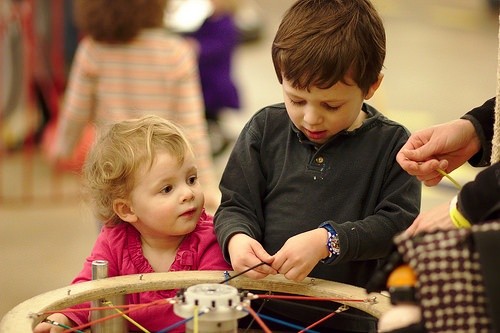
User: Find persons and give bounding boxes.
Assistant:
[393,96,500,245]
[213,0,423,333]
[33,115,234,333]
[39,0,244,174]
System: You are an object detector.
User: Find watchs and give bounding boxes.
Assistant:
[320,225,340,265]
